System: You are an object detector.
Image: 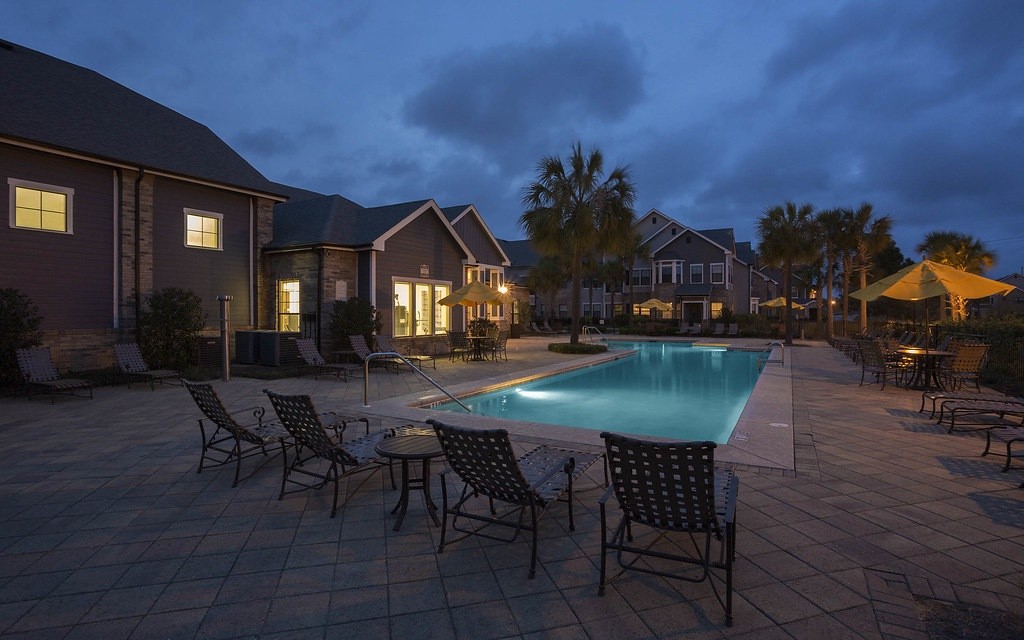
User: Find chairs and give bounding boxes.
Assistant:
[449,331,480,364]
[689,322,704,338]
[425,417,610,581]
[530,321,562,338]
[260,387,444,521]
[180,377,371,490]
[824,323,1024,491]
[112,342,184,393]
[481,328,505,360]
[13,345,93,406]
[293,336,367,384]
[446,330,472,361]
[349,334,415,377]
[711,323,725,338]
[481,329,511,364]
[373,335,436,372]
[542,322,569,337]
[638,322,690,337]
[596,430,739,628]
[726,322,800,340]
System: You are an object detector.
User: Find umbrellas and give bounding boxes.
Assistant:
[635,298,674,319]
[436,276,518,329]
[758,296,806,320]
[846,256,1021,349]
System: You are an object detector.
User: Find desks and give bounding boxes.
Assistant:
[330,348,355,378]
[896,349,957,393]
[374,433,458,531]
[455,336,494,362]
